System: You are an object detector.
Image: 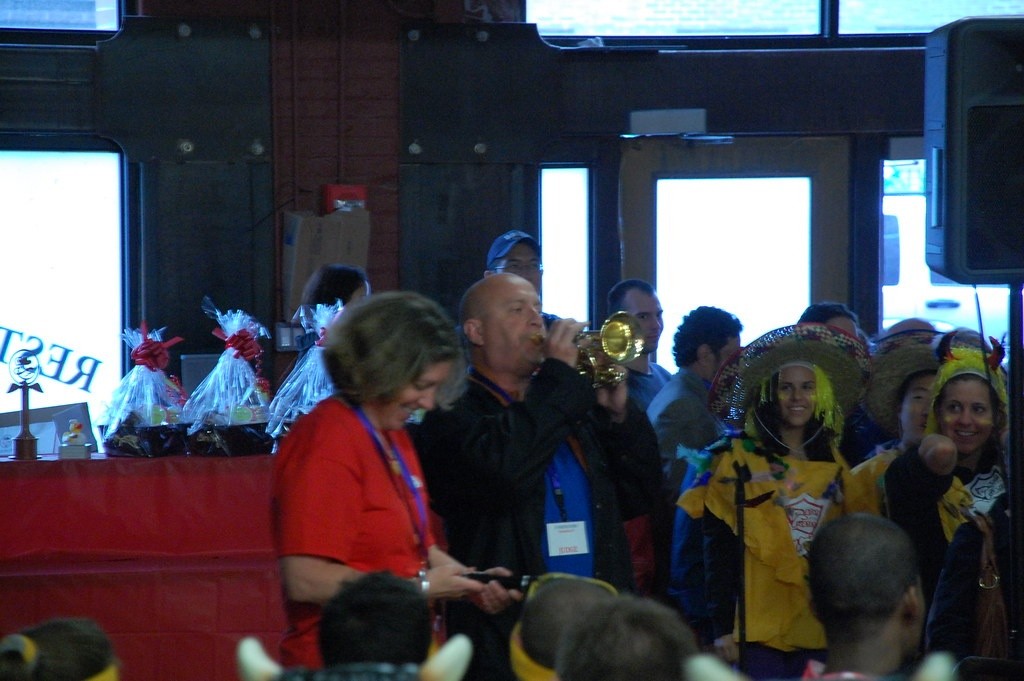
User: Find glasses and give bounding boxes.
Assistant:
[494,263,544,276]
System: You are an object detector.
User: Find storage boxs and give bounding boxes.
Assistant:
[58,444,93,459]
[0,401,273,455]
[282,209,370,318]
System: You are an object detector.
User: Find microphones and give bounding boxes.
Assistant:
[464,573,541,593]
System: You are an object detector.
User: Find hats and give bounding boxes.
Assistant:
[924,331,1010,443]
[487,230,542,266]
[707,322,871,429]
[870,329,946,436]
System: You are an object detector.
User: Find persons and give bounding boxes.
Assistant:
[269,228,1024,681]
[0,616,120,681]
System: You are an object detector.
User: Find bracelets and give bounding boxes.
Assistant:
[418,571,430,601]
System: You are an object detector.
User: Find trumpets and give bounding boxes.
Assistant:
[532,309,646,389]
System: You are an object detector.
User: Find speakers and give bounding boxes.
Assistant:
[927,15,1024,286]
